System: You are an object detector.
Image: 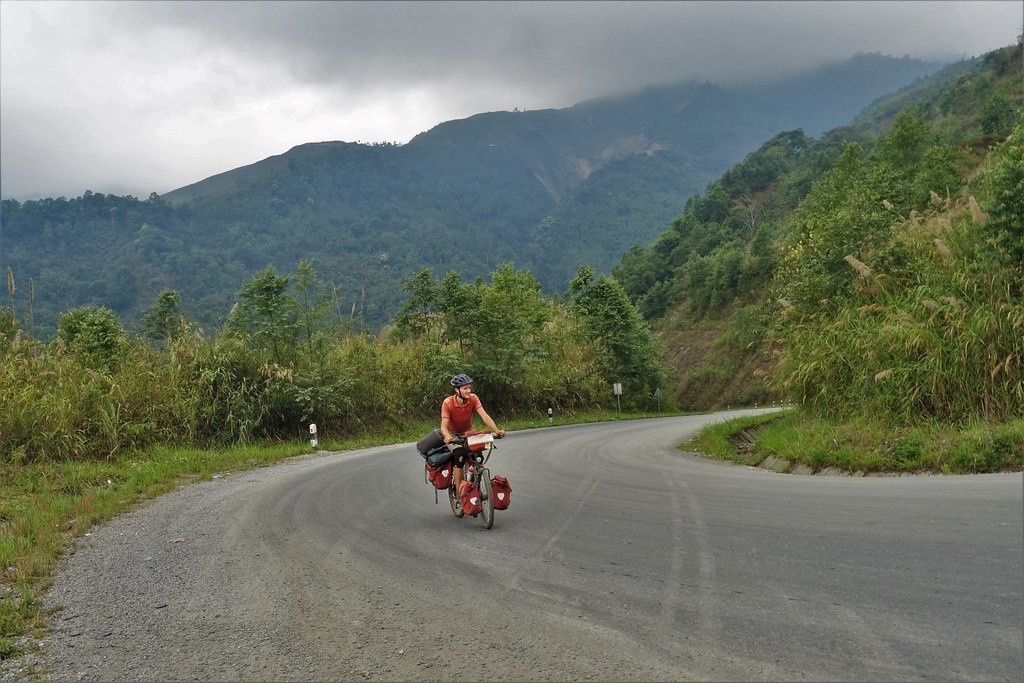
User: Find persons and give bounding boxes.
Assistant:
[440,374,505,502]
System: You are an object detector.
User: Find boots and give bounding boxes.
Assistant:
[453,467,463,498]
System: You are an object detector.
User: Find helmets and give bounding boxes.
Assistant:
[450,374,473,388]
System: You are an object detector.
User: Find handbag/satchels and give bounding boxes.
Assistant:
[491,475,512,510]
[416,431,453,490]
[457,480,483,516]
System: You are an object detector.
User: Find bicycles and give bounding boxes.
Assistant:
[448,433,504,530]
